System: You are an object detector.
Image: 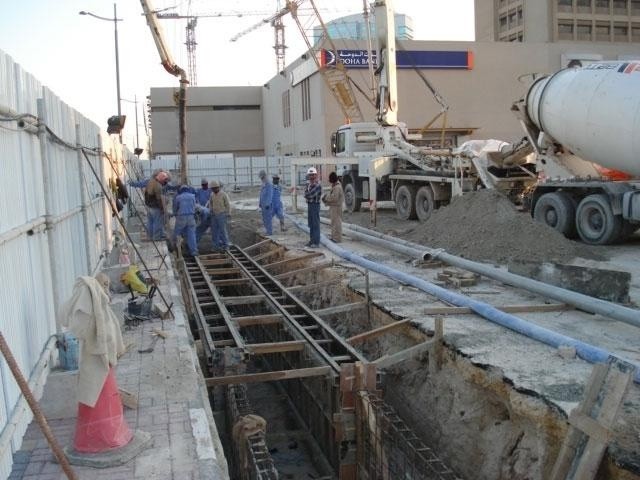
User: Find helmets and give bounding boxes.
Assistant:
[273,174,278,178]
[200,177,208,184]
[210,181,219,188]
[259,170,264,179]
[156,172,169,184]
[179,185,189,191]
[307,167,317,174]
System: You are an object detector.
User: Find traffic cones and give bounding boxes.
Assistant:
[58,277,156,470]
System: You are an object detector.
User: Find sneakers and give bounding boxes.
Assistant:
[304,243,319,248]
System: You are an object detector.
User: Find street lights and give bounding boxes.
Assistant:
[77,2,126,145]
[120,94,141,160]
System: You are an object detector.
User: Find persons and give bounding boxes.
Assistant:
[271,173,287,233]
[256,170,273,236]
[127,167,232,255]
[304,167,324,248]
[324,172,344,243]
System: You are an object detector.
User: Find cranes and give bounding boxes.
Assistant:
[229,0,305,75]
[156,0,348,86]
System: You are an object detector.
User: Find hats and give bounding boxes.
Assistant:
[329,172,336,183]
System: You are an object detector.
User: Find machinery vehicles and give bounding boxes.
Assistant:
[326,1,482,227]
[488,56,640,246]
